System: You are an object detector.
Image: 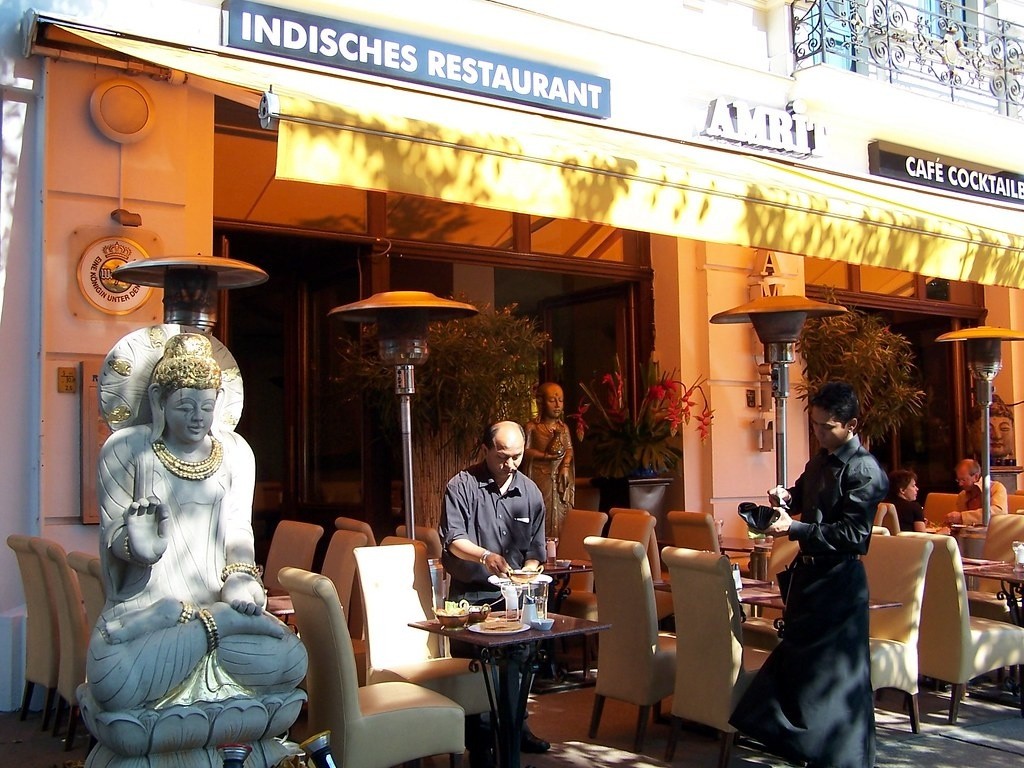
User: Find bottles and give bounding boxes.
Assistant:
[521,595,537,629]
[548,539,556,563]
[1012,540,1024,572]
[505,587,519,621]
[731,562,742,590]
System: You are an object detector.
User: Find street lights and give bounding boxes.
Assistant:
[708,295,850,492]
[933,325,1024,526]
[325,289,480,539]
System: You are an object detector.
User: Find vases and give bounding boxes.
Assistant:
[628,483,671,541]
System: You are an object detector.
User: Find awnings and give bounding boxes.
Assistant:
[17,7,1024,288]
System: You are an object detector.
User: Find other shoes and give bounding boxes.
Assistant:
[469,746,499,768]
[518,722,550,753]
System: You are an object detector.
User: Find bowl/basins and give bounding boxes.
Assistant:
[505,564,544,584]
[556,559,571,570]
[545,563,557,571]
[436,612,470,631]
[461,605,491,622]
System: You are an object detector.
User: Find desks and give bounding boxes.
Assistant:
[408,610,611,768]
[651,577,774,593]
[528,559,594,694]
[964,562,1024,710]
[737,585,903,611]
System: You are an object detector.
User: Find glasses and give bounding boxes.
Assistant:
[956,474,975,484]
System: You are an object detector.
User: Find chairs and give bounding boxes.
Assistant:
[658,548,771,768]
[965,514,1024,623]
[666,510,758,619]
[583,536,679,754]
[7,515,500,768]
[574,477,601,511]
[872,502,901,536]
[610,509,672,590]
[554,510,608,591]
[922,493,961,528]
[896,531,1024,726]
[869,535,933,733]
[552,515,656,621]
[1007,494,1024,514]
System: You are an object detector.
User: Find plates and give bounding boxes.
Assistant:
[469,623,530,635]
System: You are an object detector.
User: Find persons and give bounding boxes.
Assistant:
[965,393,1014,463]
[943,458,1008,527]
[729,385,886,768]
[522,380,575,549]
[80,322,310,708]
[887,469,951,535]
[437,420,552,768]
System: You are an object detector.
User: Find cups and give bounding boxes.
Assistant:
[529,584,548,619]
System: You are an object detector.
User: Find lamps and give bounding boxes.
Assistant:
[709,294,849,488]
[110,208,143,227]
[935,326,1024,528]
[106,252,267,330]
[326,291,478,539]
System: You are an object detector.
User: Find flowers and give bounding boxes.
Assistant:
[563,349,715,477]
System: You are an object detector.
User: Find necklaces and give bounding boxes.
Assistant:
[152,434,224,480]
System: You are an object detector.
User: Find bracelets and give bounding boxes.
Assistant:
[221,562,264,584]
[479,551,490,564]
[124,535,163,567]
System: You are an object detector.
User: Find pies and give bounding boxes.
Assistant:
[480,621,523,632]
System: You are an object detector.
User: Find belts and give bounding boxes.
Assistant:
[798,554,860,566]
